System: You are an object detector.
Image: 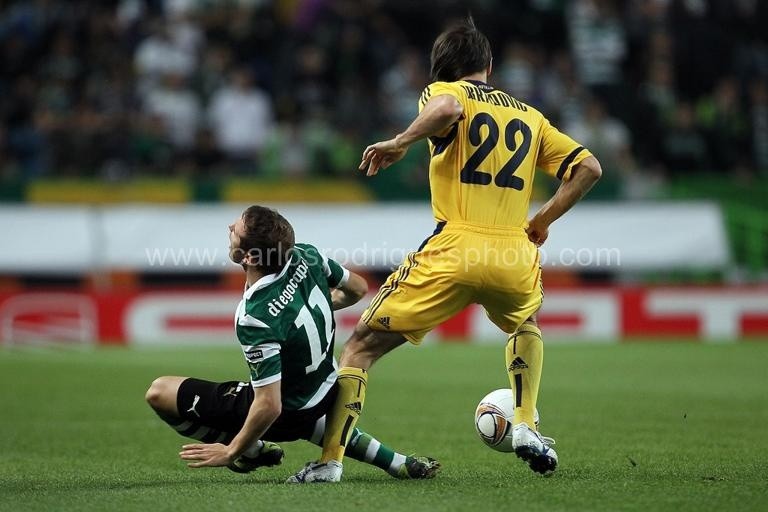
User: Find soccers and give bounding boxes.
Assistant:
[473,389,540,454]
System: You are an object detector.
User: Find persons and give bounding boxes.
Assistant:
[146,207,441,475]
[284,20,603,481]
[1,1,768,200]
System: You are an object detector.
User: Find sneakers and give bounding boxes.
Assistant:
[228,440,285,473]
[511,422,559,474]
[396,456,442,479]
[284,460,343,483]
